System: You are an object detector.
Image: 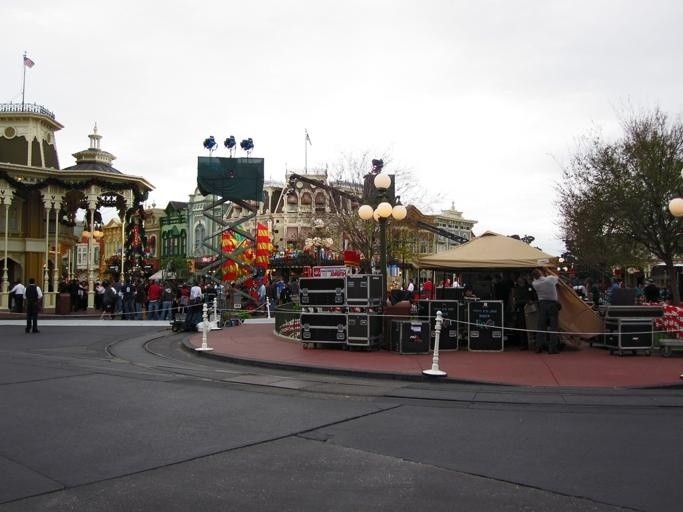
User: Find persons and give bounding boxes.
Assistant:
[5,270,299,334]
[386,264,672,354]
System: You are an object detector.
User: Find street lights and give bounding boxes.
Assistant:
[358,172,408,307]
[78,230,104,291]
[305,219,334,266]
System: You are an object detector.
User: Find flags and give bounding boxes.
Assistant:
[24,57,34,68]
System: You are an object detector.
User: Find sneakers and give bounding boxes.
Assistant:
[535,349,542,353]
[25,329,41,334]
[549,349,560,354]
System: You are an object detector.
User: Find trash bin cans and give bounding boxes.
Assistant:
[57,293,70,314]
